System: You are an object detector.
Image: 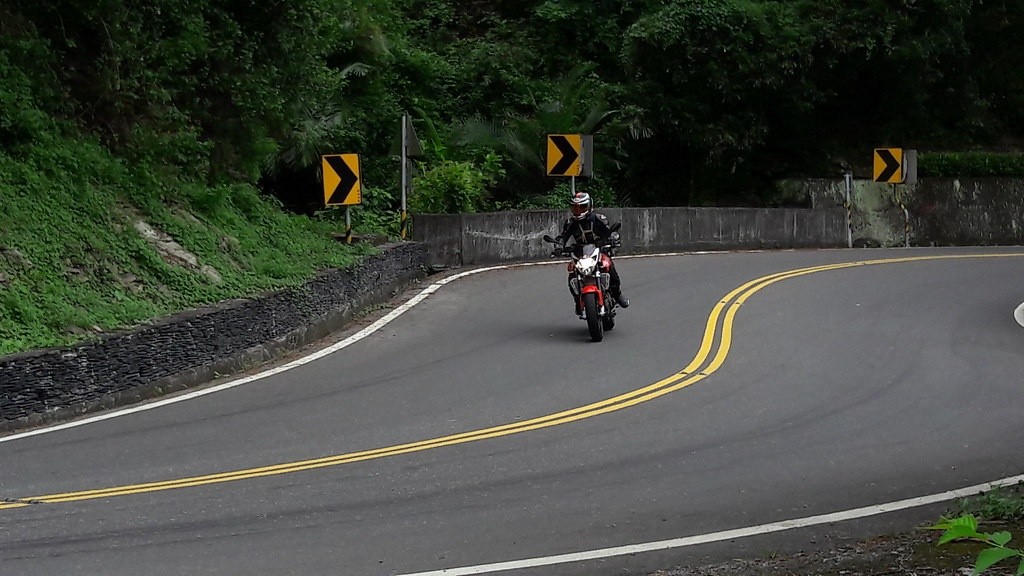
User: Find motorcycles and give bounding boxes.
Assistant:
[543,222,622,342]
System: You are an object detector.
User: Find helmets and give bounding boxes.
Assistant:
[569,192,590,220]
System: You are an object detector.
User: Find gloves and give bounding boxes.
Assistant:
[608,231,621,243]
[550,248,562,258]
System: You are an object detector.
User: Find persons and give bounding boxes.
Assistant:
[554,192,628,315]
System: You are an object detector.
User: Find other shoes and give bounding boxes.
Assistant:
[576,303,583,316]
[614,293,628,308]
